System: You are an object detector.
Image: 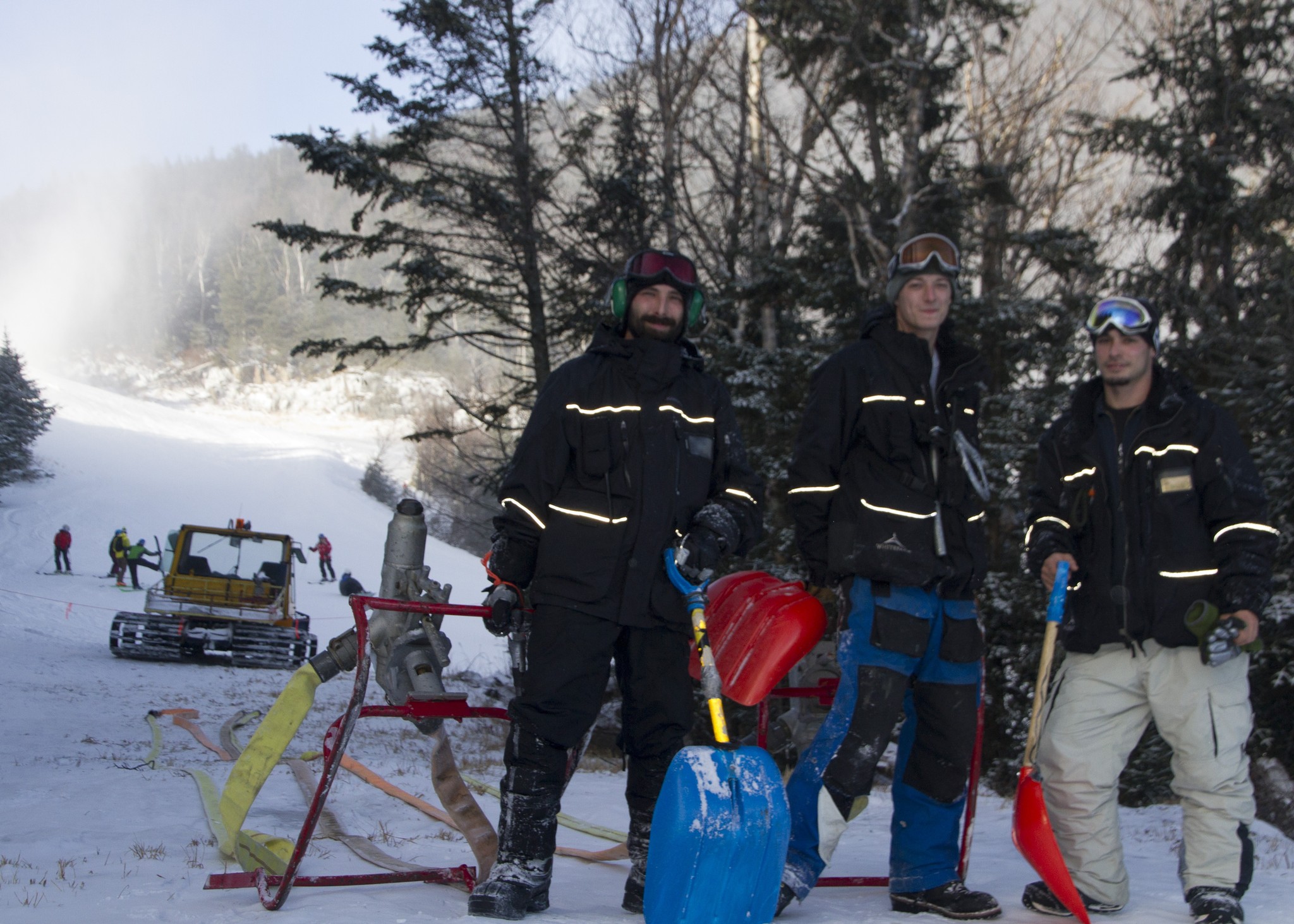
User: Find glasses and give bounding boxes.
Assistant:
[1084,297,1159,334]
[624,248,697,298]
[888,233,961,281]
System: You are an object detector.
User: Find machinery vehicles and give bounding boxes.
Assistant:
[109,518,318,669]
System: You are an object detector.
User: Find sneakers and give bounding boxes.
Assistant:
[1022,876,1125,917]
[1184,883,1246,924]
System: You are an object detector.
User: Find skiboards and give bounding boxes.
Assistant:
[99,582,144,588]
[120,588,146,592]
[35,571,83,576]
[92,575,131,579]
[307,581,330,584]
[153,535,165,578]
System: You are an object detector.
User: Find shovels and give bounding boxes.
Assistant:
[1010,558,1094,923]
[639,547,796,923]
[688,569,828,707]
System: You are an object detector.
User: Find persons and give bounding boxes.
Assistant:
[775,231,1002,921]
[109,529,121,577]
[308,534,337,582]
[1022,294,1280,924]
[339,572,366,596]
[124,539,161,590]
[112,528,130,586]
[469,248,761,920]
[54,524,72,573]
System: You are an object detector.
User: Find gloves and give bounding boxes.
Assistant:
[480,583,523,637]
[1184,599,1263,668]
[675,524,726,584]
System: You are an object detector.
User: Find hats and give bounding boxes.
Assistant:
[886,257,956,305]
[621,266,689,323]
[1082,296,1160,352]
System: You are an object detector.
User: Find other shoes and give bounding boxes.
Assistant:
[467,868,550,921]
[620,867,648,915]
[889,880,1002,921]
[773,879,798,918]
